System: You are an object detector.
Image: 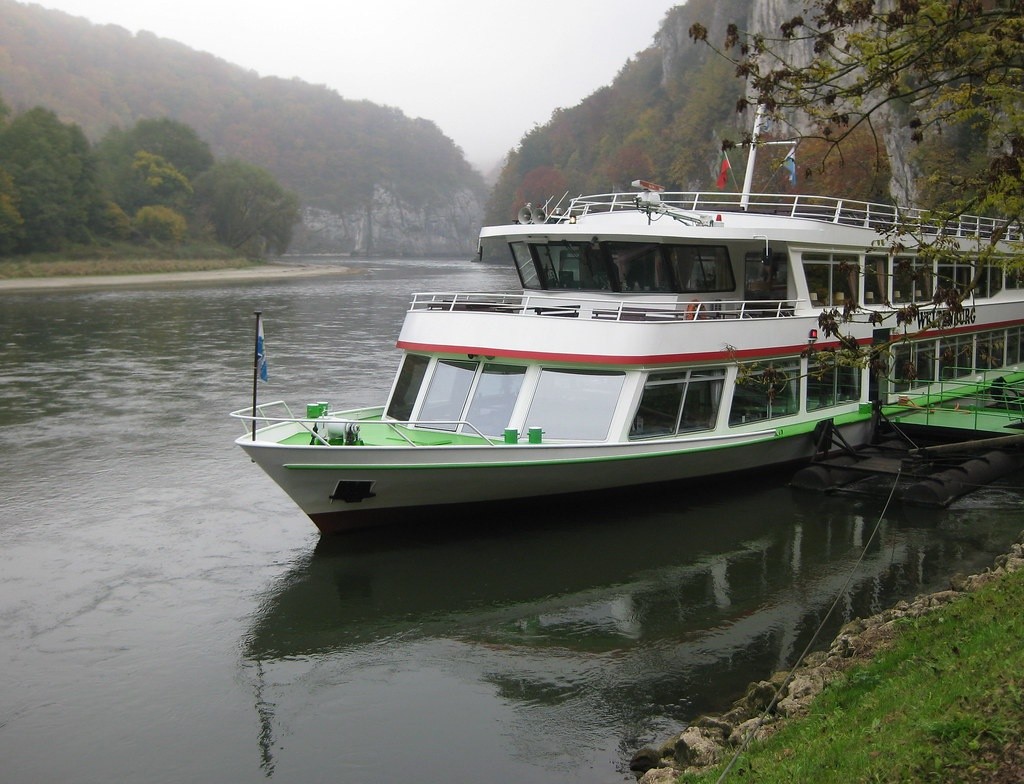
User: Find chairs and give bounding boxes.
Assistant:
[814,288,829,306]
[714,299,726,320]
[868,288,882,304]
[558,270,575,289]
[919,289,931,301]
[775,210,1020,241]
[673,258,698,290]
[896,290,911,302]
[836,287,851,306]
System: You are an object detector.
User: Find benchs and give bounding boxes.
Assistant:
[732,394,851,416]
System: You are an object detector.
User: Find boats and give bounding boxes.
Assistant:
[228,98,1024,534]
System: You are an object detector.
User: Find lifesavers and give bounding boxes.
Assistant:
[687,300,708,320]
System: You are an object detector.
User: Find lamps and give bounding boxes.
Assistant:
[915,290,922,303]
[866,291,874,304]
[836,292,844,306]
[809,292,818,307]
[894,290,900,303]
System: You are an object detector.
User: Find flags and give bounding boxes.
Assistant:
[781,146,797,190]
[257,314,268,385]
[717,152,730,189]
